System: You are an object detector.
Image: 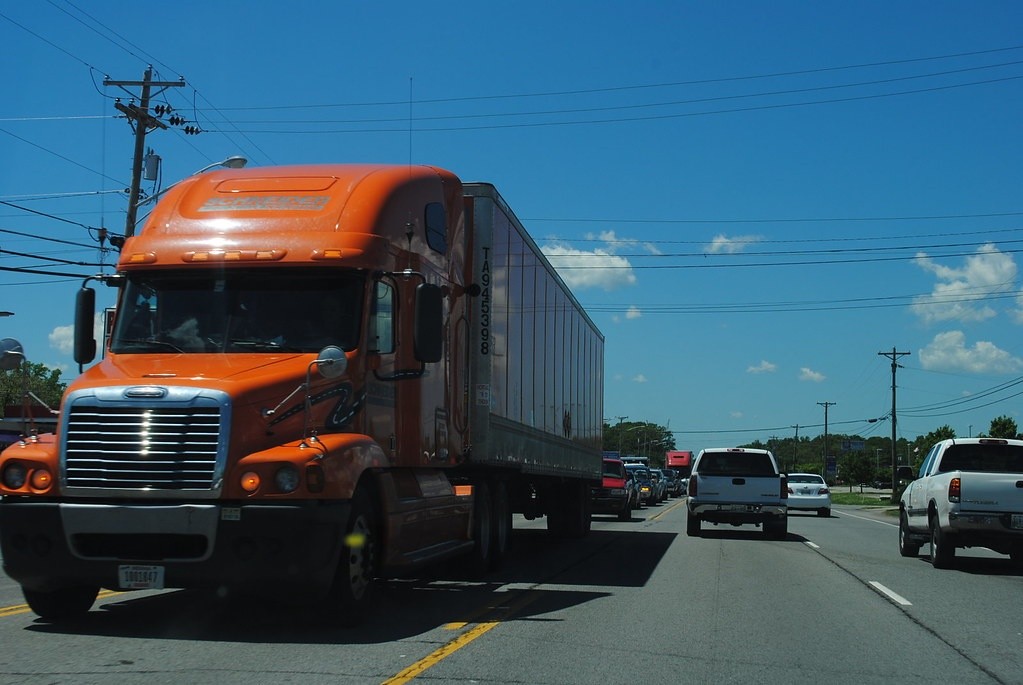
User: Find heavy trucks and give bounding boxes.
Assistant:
[0,146,608,619]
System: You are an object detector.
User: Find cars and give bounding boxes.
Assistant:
[685,447,790,538]
[779,471,831,518]
[581,442,693,523]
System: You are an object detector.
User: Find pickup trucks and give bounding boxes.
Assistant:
[894,434,1023,574]
